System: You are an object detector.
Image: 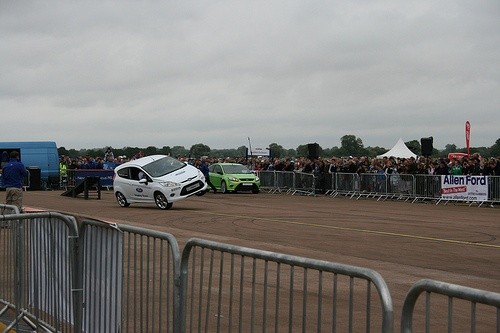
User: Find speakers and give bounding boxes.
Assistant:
[421,138,433,156]
[309,144,320,158]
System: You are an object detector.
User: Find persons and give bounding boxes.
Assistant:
[1,151,27,229]
[314,156,436,203]
[433,153,500,206]
[59,147,145,184]
[231,156,315,193]
[178,155,230,195]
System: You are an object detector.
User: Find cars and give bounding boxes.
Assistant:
[208,162,261,194]
[113,154,208,210]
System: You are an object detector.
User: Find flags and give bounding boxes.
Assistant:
[466,121,470,154]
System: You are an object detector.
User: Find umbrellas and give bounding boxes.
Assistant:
[376,138,420,160]
